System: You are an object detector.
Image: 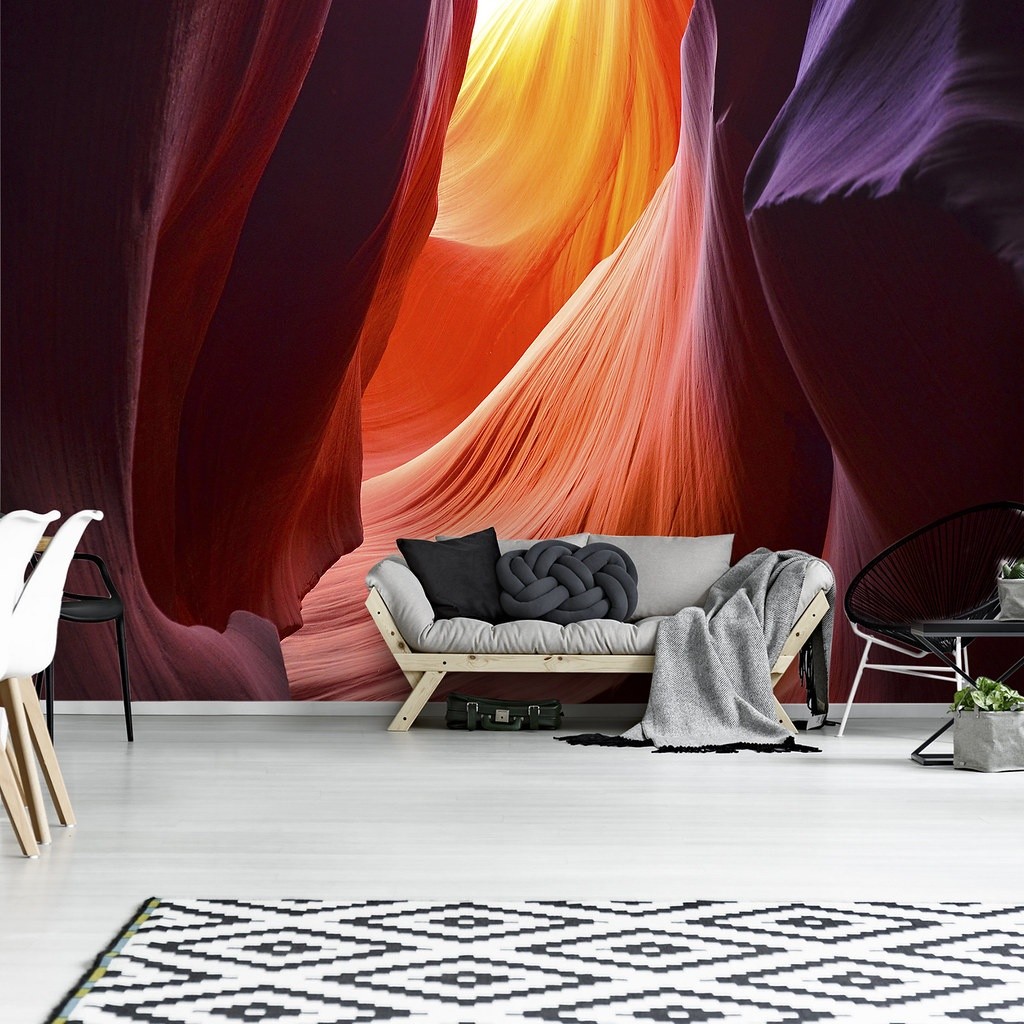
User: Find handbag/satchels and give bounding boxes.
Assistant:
[993,578,1024,621]
[445,691,565,731]
[952,687,1024,772]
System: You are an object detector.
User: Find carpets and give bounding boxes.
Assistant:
[46,898,1024,1024]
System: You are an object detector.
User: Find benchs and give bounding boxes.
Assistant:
[364,554,830,730]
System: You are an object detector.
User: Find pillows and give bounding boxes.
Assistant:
[435,533,589,557]
[396,526,510,626]
[588,534,734,620]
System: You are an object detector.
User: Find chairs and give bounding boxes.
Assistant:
[9,509,104,828]
[837,505,1024,739]
[0,509,61,860]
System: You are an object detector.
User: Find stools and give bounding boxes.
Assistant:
[23,536,135,747]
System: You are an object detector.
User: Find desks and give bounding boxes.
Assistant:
[910,620,1024,765]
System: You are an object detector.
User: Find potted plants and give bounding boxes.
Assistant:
[998,560,1024,623]
[946,676,1024,774]
